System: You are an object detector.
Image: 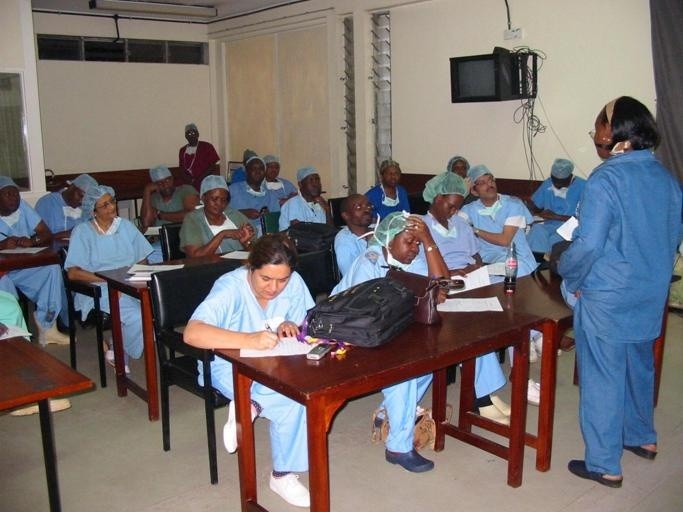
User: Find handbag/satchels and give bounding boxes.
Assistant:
[385,269,447,327]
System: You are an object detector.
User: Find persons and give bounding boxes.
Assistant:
[179,230,318,508]
[0,176,78,347]
[328,209,450,473]
[31,172,99,335]
[136,147,324,263]
[61,183,157,380]
[422,155,587,427]
[334,158,409,279]
[555,96,683,488]
[178,123,222,192]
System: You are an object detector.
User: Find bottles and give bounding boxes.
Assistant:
[502,241,518,287]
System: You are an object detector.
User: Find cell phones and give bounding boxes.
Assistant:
[306,342,332,360]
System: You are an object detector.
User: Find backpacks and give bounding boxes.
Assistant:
[303,275,417,349]
[284,220,341,254]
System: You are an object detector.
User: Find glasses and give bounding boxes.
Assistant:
[587,127,613,142]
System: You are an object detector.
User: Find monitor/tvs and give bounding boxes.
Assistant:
[448,47,513,104]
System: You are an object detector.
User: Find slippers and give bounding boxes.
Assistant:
[620,441,658,460]
[568,460,624,488]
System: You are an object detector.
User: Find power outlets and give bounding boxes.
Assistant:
[511,27,522,39]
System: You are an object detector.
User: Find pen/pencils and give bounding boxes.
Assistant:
[0,231,8,237]
[265,324,282,344]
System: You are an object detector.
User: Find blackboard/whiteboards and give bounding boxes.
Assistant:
[37,60,213,177]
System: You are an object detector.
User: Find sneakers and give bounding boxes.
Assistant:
[9,397,76,416]
[489,393,511,415]
[527,377,542,406]
[223,398,256,454]
[106,351,132,377]
[38,324,79,347]
[385,447,435,473]
[268,470,310,508]
[478,403,512,426]
[529,337,539,363]
[533,334,563,359]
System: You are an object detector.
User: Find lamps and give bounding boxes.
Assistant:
[113,14,124,44]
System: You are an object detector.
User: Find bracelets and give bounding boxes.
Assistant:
[31,235,40,247]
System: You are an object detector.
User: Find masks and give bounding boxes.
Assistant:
[185,146,199,155]
[479,200,504,217]
[431,219,457,240]
[1,209,21,227]
[387,252,417,271]
[266,181,285,190]
[382,195,400,207]
[105,217,122,235]
[62,205,83,219]
[551,183,569,199]
[245,187,265,197]
[208,218,238,237]
